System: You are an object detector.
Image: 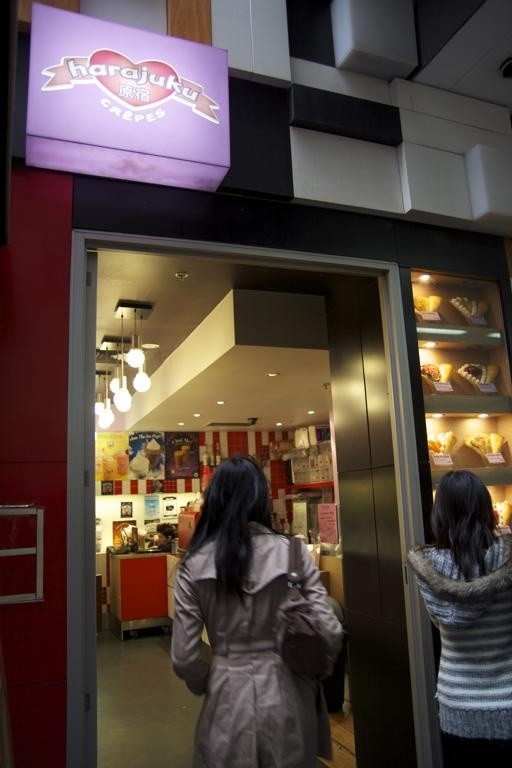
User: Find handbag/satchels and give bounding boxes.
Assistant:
[274,536,347,681]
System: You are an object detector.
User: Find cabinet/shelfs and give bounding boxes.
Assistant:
[408,266,512,531]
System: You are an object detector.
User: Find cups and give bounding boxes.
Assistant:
[174,446,191,470]
[130,445,163,478]
[97,455,128,482]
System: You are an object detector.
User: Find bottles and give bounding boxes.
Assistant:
[203,442,222,466]
[171,537,179,555]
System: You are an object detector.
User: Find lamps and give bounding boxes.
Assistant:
[94,299,153,428]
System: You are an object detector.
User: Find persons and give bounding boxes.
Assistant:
[406,468,511,768]
[165,452,345,767]
[156,522,176,552]
